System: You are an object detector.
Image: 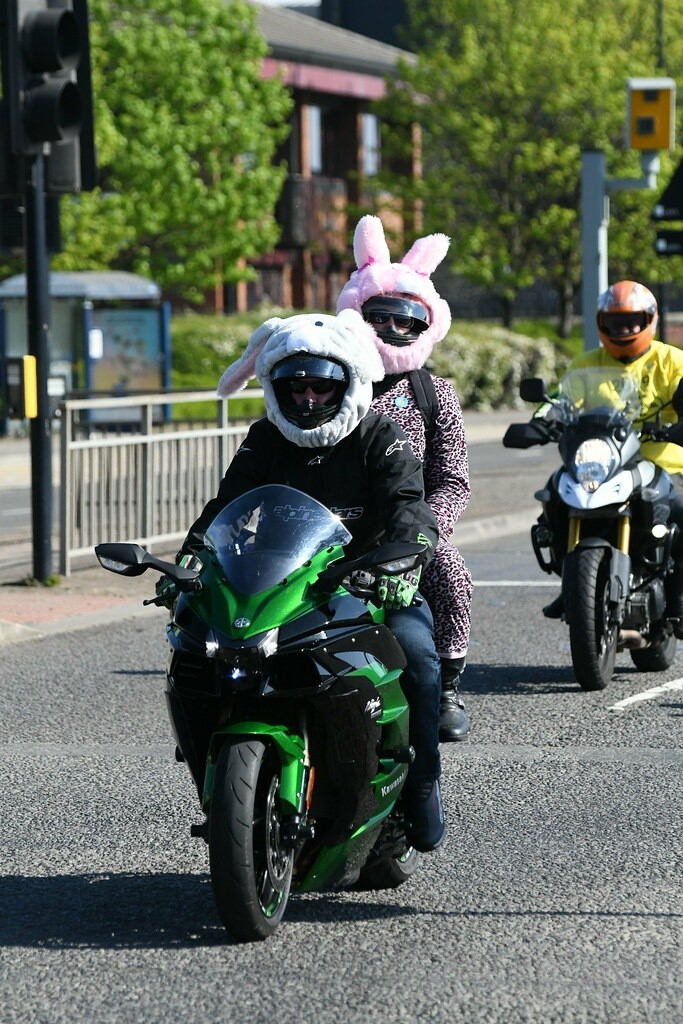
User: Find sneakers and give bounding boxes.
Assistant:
[403,773,444,853]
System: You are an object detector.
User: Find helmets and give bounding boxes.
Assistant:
[361,291,432,348]
[269,352,349,431]
[594,280,658,359]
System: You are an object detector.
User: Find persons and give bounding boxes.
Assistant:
[325,212,478,746]
[523,277,683,641]
[151,302,447,850]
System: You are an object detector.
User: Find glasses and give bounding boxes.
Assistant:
[361,308,416,329]
[283,376,340,395]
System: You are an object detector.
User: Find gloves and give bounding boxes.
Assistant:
[376,532,435,611]
[154,551,193,613]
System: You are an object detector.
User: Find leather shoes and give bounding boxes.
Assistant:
[440,675,469,742]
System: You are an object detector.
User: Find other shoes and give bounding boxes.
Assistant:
[542,590,564,619]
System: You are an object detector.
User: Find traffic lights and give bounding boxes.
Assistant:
[1,1,86,157]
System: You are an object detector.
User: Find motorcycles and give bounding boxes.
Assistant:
[88,484,421,938]
[505,367,683,697]
